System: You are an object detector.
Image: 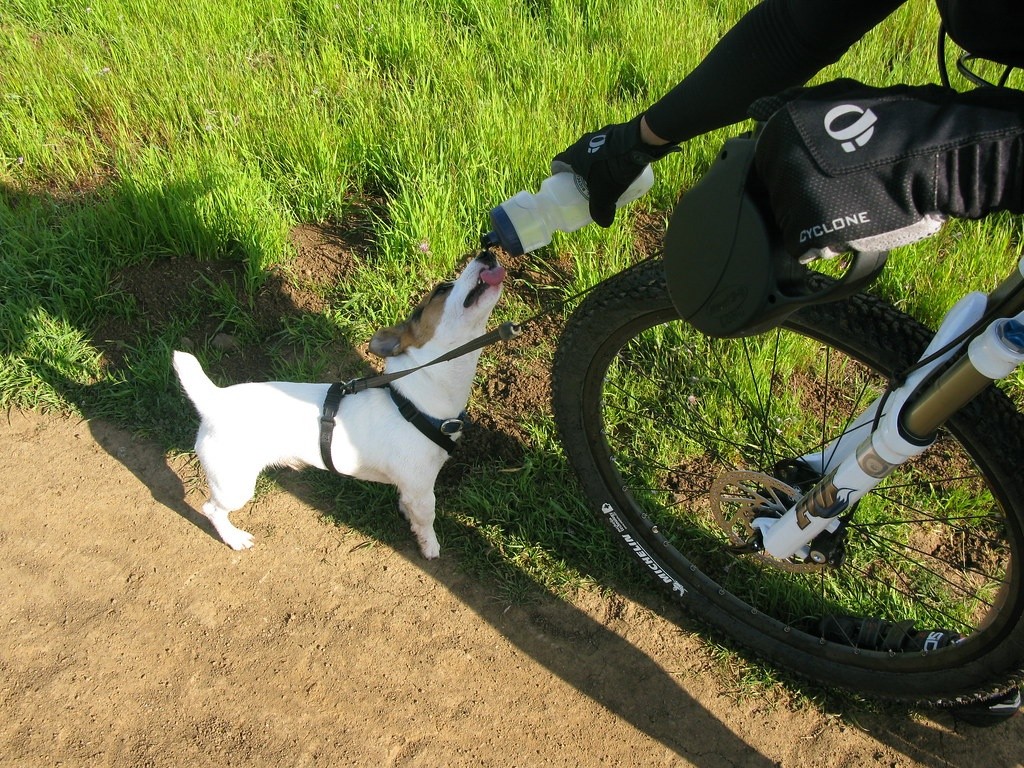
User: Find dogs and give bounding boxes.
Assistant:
[171,252,508,559]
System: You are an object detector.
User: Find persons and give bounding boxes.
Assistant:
[549,0,1024,729]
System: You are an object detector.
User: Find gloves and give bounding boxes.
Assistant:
[549,110,683,228]
[743,78,1024,265]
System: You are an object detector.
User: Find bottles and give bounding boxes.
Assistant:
[479,166,656,258]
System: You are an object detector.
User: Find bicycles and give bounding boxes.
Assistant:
[551,0,1023,718]
